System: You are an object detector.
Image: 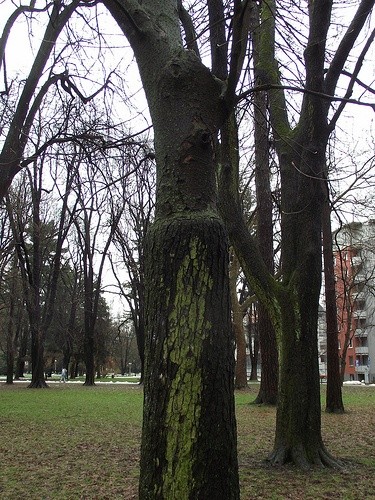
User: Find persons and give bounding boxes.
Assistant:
[60,367,68,383]
